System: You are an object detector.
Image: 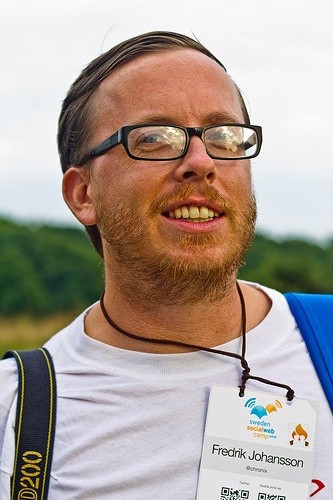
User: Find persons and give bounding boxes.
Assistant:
[0,29,330,500]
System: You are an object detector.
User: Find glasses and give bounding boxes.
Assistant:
[72,119,265,166]
